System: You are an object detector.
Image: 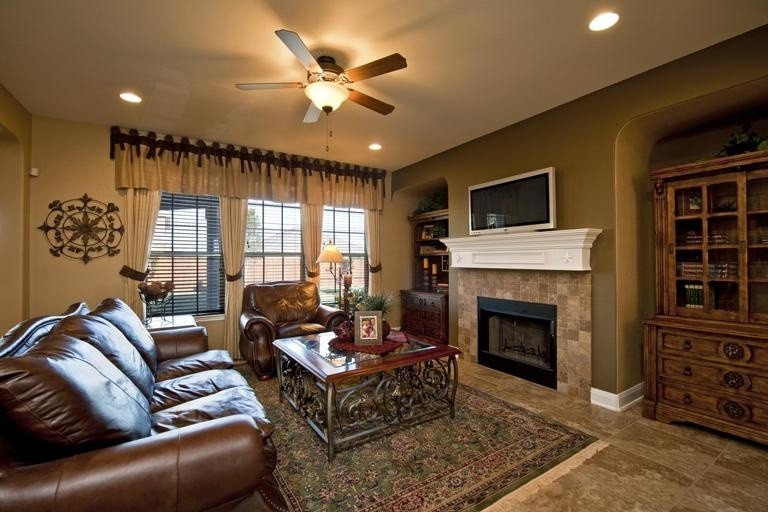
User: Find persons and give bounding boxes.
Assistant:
[360,317,376,338]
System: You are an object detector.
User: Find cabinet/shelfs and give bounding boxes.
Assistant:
[399,288,447,344]
[144,314,197,331]
[640,313,767,451]
[647,146,767,323]
[438,228,602,273]
[406,207,448,291]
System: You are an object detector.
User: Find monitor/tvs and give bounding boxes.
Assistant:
[468,167,556,235]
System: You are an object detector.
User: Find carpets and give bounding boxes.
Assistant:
[233,360,609,511]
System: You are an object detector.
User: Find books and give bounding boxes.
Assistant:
[707,231,729,244]
[707,262,737,278]
[758,233,768,244]
[682,283,704,308]
[678,262,704,278]
[676,189,704,217]
[685,233,703,243]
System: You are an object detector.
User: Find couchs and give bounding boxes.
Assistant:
[0,297,275,511]
[237,280,344,382]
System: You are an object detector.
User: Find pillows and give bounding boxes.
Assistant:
[52,310,156,406]
[276,320,324,336]
[89,296,159,375]
[0,333,152,452]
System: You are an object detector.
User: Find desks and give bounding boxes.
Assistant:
[268,331,464,463]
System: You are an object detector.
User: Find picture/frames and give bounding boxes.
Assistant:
[352,310,383,347]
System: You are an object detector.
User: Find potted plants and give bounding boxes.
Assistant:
[359,294,388,338]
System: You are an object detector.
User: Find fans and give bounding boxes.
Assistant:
[230,26,406,123]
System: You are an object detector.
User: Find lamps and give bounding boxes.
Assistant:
[303,80,349,117]
[315,244,344,309]
